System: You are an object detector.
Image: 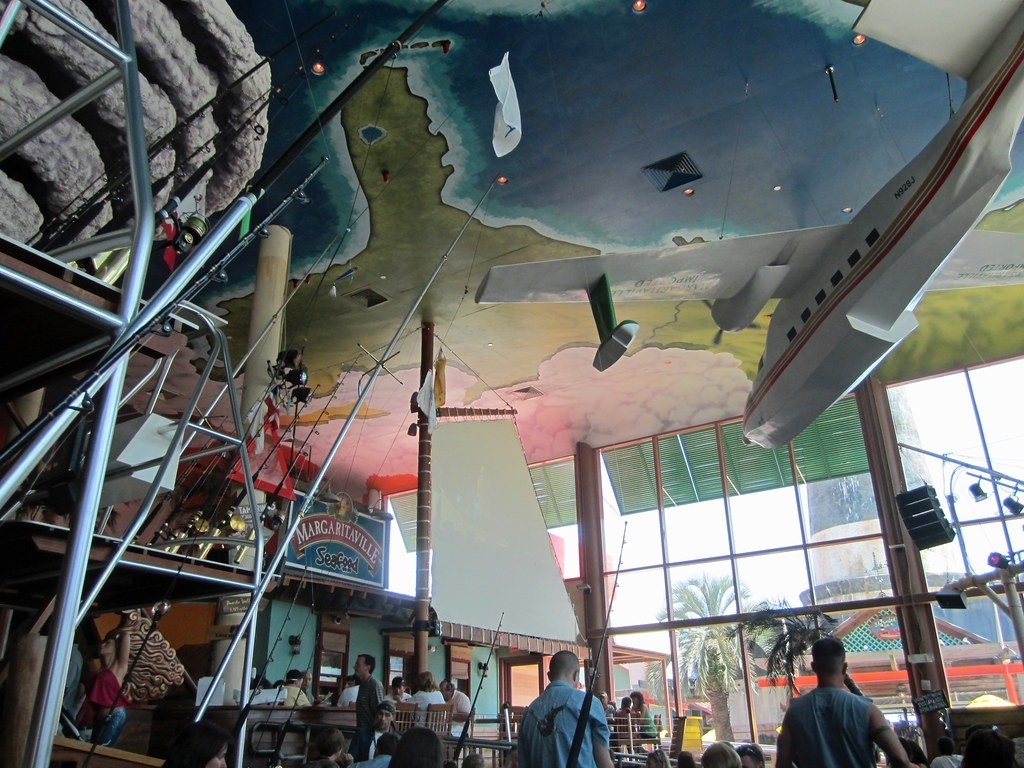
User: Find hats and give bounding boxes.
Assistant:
[598,691,607,697]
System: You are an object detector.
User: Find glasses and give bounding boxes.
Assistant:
[740,744,761,761]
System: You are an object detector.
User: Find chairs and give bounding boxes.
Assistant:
[499,705,528,768]
[393,703,419,732]
[452,706,476,761]
[425,703,454,763]
[606,713,663,762]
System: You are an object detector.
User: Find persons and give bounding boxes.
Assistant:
[599,691,765,768]
[88,631,131,747]
[164,654,485,768]
[775,638,919,768]
[517,650,614,768]
[886,723,1024,768]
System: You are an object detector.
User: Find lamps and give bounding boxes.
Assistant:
[968,483,988,502]
[935,588,967,610]
[987,552,1008,569]
[276,349,315,404]
[1003,498,1024,516]
[407,423,420,437]
